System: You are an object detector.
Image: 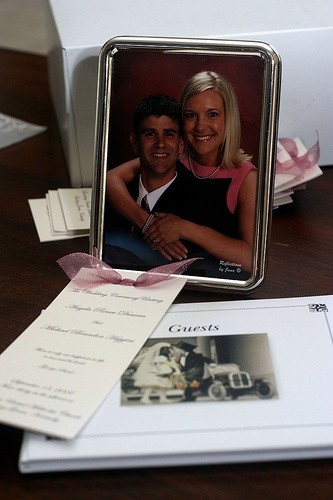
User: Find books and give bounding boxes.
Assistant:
[19,295,333,473]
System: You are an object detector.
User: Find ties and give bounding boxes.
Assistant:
[141,198,150,215]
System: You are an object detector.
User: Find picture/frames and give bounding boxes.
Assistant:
[87,34,282,290]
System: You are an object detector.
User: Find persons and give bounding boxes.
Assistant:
[131,341,217,406]
[106,70,257,281]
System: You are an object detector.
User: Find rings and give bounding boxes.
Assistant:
[153,238,158,243]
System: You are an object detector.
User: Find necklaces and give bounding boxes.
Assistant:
[188,153,222,179]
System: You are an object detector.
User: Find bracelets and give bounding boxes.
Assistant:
[141,214,155,233]
